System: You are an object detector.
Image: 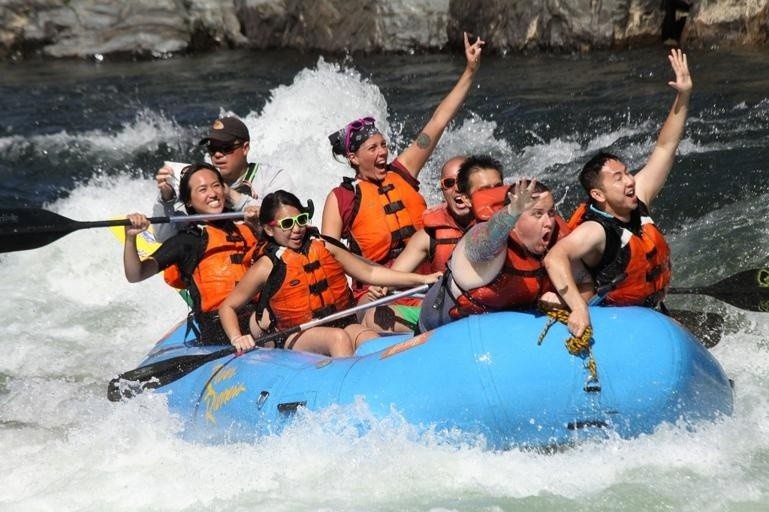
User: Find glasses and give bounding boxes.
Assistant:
[269,213,310,231]
[178,166,193,180]
[344,117,375,153]
[440,177,456,190]
[207,143,241,155]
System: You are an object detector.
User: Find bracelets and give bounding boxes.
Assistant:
[229,334,242,347]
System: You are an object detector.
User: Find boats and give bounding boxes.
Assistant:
[134,303,734,453]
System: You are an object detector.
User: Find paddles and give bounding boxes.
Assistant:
[388,288,722,348]
[659,268,768,313]
[106,282,438,403]
[0,206,254,253]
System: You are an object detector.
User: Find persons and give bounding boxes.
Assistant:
[121,158,278,348]
[151,113,307,246]
[540,47,695,339]
[365,153,478,305]
[318,31,490,333]
[457,154,506,214]
[214,188,446,360]
[410,173,596,339]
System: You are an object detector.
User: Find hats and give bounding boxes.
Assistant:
[199,116,250,145]
